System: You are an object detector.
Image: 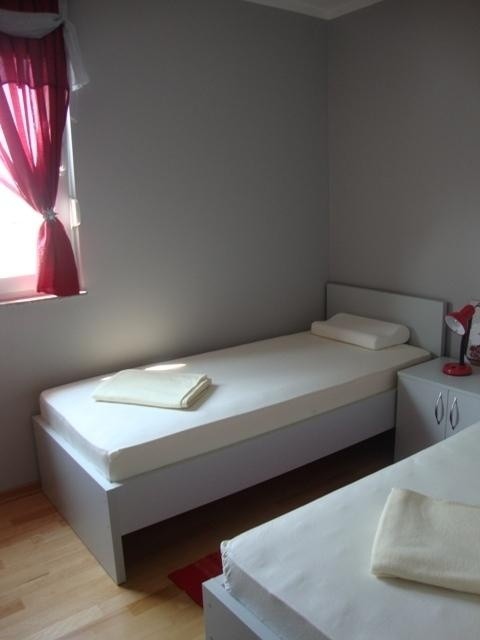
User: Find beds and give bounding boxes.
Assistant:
[31,281,451,585]
[202,422,480,640]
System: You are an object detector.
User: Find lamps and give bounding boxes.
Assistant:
[443,305,475,376]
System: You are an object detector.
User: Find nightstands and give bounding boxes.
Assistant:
[396,358,480,462]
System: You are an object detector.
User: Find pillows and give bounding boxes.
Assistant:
[311,312,411,351]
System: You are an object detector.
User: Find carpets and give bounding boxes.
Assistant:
[168,550,223,609]
